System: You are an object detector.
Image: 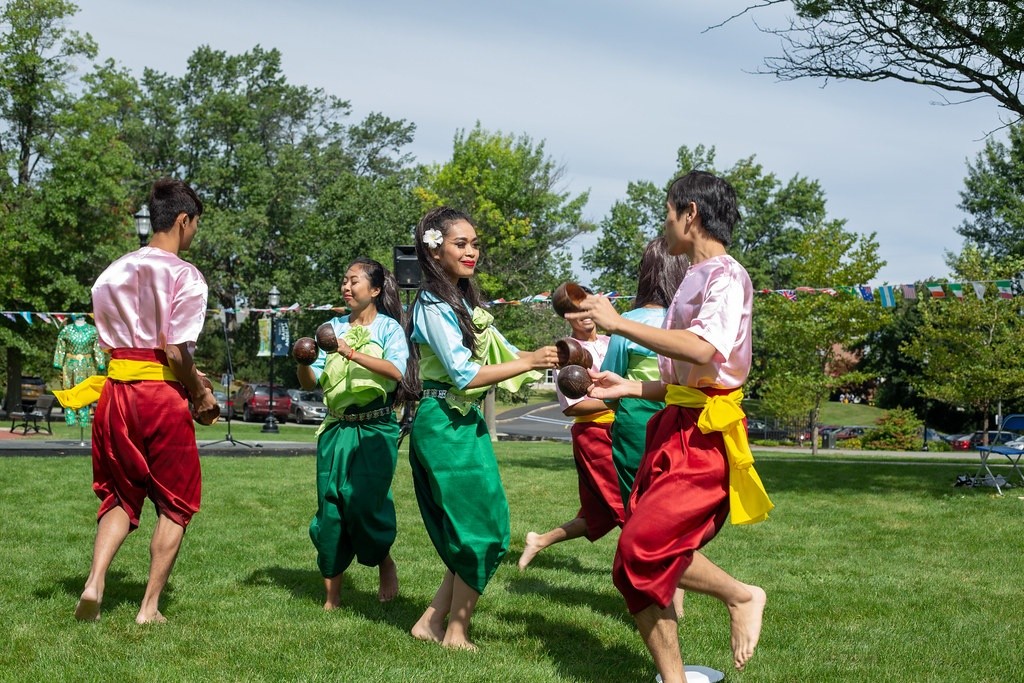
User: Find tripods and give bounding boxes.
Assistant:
[200,321,256,450]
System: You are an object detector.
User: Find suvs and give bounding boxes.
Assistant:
[229,383,328,425]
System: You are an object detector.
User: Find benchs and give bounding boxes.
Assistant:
[10,393,56,435]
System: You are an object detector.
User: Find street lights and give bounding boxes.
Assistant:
[261,285,279,434]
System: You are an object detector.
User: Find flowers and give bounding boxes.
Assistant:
[422,227,444,250]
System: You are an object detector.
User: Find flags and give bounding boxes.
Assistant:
[486,290,622,306]
[3,302,332,324]
[756,279,1024,307]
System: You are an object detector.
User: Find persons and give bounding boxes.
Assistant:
[53,317,106,426]
[563,170,774,683]
[518,287,627,573]
[409,206,560,652]
[298,257,415,610]
[75,176,220,628]
[600,236,690,617]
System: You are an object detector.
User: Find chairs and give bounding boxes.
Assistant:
[970,414,1024,496]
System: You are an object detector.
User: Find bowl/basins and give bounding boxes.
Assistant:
[293,337,319,366]
[557,365,593,399]
[191,404,221,426]
[580,349,593,369]
[552,282,587,318]
[556,338,583,369]
[316,323,338,351]
[184,376,213,403]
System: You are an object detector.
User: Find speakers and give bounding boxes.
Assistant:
[394,245,423,290]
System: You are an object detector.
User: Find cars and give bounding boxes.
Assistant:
[747,419,870,441]
[211,393,233,422]
